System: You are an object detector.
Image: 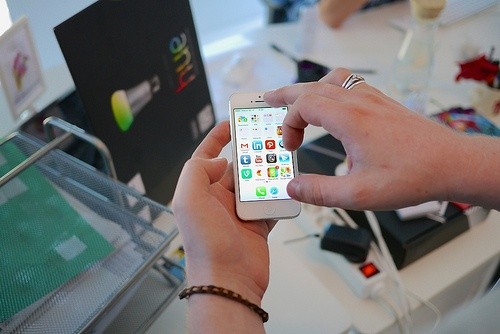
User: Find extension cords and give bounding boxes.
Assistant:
[306,228,387,299]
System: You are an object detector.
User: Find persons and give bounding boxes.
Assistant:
[172,66,500,334]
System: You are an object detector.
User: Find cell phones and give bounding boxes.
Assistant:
[229,93,301,221]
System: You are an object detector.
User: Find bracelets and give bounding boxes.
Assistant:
[178,285,268,323]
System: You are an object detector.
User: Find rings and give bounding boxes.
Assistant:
[341,73,365,90]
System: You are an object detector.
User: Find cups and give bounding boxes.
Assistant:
[397,17,441,67]
[410,0,447,17]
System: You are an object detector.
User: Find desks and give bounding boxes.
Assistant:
[44,0,500,334]
